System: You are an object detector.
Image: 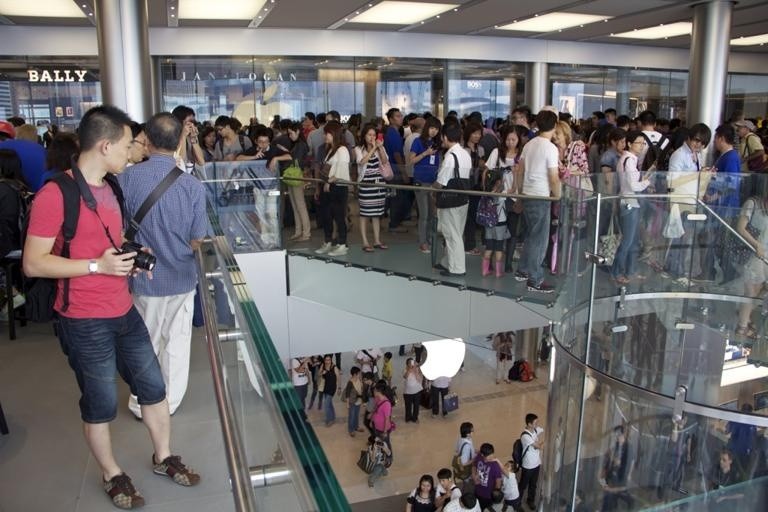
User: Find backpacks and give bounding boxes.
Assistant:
[1,179,35,232]
[509,358,534,382]
[512,428,537,472]
[476,196,504,228]
[639,132,668,195]
[22,172,124,324]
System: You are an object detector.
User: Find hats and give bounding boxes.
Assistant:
[0,120,16,138]
[735,120,755,131]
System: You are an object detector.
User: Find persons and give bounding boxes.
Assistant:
[0,102,767,331]
[281,330,766,511]
[20,103,201,511]
[118,110,210,421]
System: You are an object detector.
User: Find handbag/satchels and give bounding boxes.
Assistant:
[222,185,255,206]
[357,437,392,475]
[477,168,504,192]
[341,388,358,403]
[748,154,768,172]
[444,393,459,412]
[380,161,393,180]
[436,178,471,208]
[452,456,473,480]
[715,218,761,265]
[566,164,594,199]
[282,168,304,186]
[318,379,325,392]
[598,235,622,266]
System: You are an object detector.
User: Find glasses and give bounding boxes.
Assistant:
[215,126,224,131]
[693,138,705,145]
[131,139,145,147]
[512,115,522,120]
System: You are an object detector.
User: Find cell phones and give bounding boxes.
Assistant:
[708,165,716,173]
[378,133,382,141]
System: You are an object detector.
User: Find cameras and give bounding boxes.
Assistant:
[376,441,384,446]
[188,123,198,134]
[119,242,156,270]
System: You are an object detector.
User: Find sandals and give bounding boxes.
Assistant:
[735,323,762,340]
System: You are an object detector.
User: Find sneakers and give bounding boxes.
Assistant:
[103,471,145,509]
[153,452,200,486]
[355,427,364,432]
[350,432,355,436]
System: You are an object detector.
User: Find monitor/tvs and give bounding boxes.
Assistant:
[752,390,768,410]
[718,399,738,422]
